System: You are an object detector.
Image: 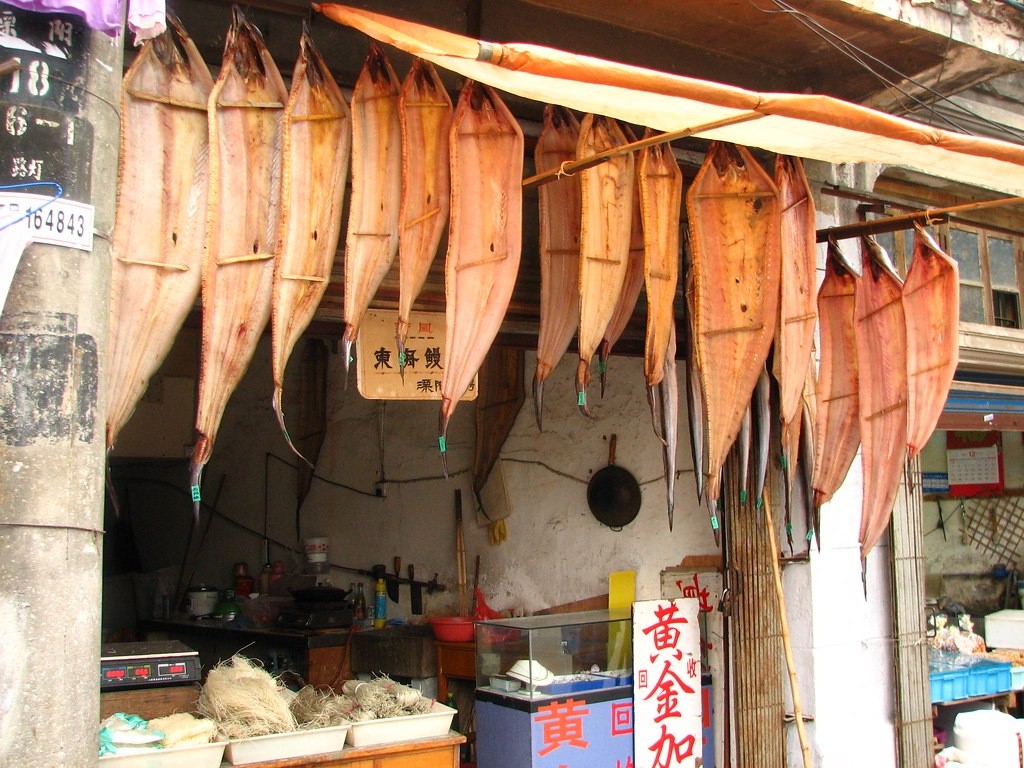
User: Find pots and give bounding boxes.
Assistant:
[586,433,641,532]
[185,582,219,617]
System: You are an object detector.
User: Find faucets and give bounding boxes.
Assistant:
[428,572,440,588]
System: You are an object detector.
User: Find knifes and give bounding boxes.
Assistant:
[387,556,401,603]
[408,564,423,615]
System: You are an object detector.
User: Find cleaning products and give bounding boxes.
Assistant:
[374,577,387,629]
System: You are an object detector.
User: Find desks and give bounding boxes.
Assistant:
[139,619,356,695]
[940,692,1008,710]
[927,659,1024,703]
[433,640,608,703]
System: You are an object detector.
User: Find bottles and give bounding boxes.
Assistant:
[348,582,368,620]
[374,578,387,628]
[260,561,283,595]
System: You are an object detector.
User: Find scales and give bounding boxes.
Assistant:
[101,640,201,694]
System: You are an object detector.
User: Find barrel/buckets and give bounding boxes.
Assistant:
[303,537,328,563]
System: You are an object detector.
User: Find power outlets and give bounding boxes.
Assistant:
[375,481,388,497]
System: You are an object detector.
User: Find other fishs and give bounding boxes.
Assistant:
[104,5,963,603]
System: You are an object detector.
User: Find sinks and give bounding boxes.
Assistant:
[350,622,437,679]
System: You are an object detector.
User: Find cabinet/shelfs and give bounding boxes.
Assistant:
[474,606,716,768]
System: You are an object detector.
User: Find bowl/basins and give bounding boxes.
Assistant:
[428,616,475,642]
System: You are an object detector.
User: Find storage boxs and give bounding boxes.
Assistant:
[342,697,458,746]
[538,673,620,695]
[99,734,229,768]
[984,608,1024,649]
[590,668,632,685]
[350,624,434,678]
[224,717,351,766]
